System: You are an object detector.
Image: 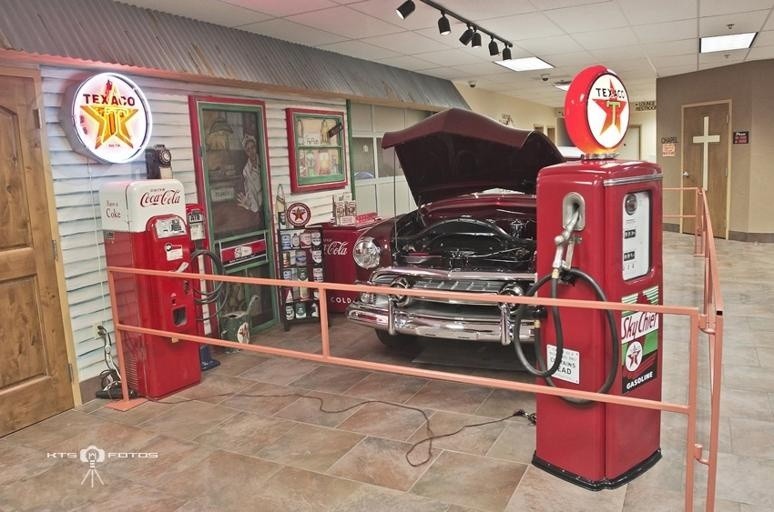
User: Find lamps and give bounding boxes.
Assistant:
[396,0,513,61]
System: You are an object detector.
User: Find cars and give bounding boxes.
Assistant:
[345,110,585,349]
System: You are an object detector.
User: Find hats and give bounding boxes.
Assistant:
[242,135,256,147]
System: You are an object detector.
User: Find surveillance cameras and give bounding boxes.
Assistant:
[541,74,550,81]
[469,81,476,87]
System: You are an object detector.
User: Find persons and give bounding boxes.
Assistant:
[233,133,266,232]
